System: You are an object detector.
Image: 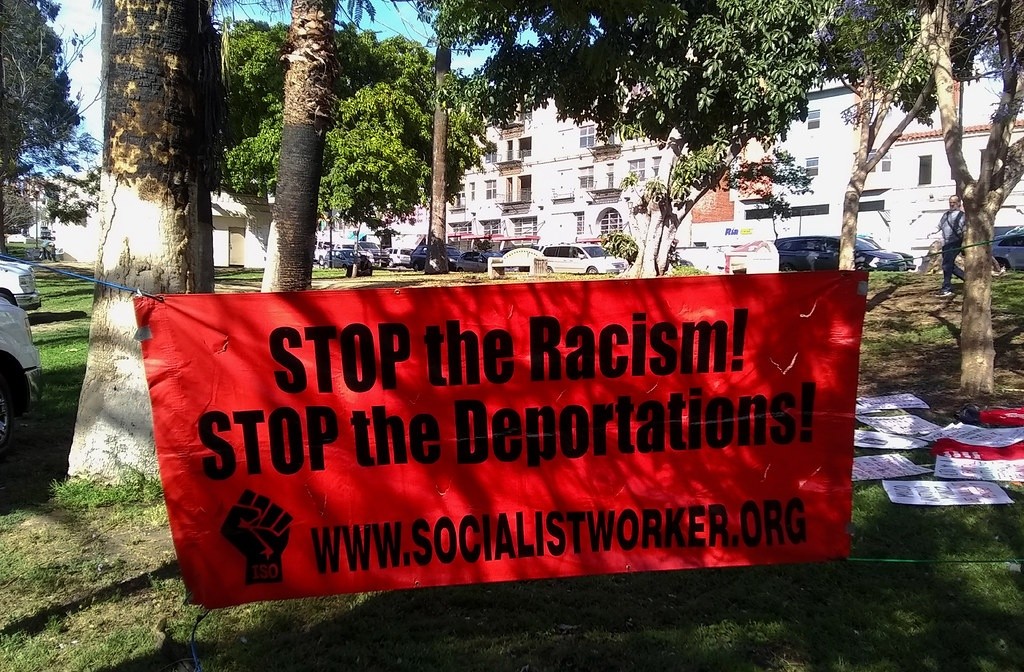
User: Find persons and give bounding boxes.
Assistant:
[927,195,966,297]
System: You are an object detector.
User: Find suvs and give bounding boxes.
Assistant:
[409,244,462,272]
[541,244,629,274]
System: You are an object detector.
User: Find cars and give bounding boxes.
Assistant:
[387,248,413,268]
[860,237,916,271]
[456,250,520,274]
[0,296,41,462]
[323,249,362,268]
[774,235,907,271]
[0,259,42,312]
[314,237,390,268]
[992,226,1024,271]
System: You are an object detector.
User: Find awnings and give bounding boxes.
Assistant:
[499,221,545,240]
[576,221,629,242]
[449,219,505,239]
[348,234,366,240]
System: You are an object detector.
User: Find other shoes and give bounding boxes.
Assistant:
[934,290,951,297]
